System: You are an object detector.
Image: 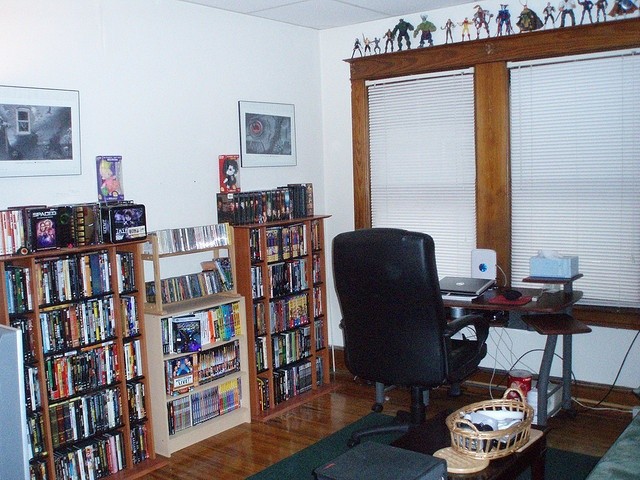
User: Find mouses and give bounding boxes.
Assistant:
[504,290,522,300]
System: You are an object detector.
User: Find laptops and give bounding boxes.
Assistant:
[440,277,495,296]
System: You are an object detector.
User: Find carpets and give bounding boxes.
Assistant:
[242,410,602,479]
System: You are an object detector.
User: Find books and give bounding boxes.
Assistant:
[257,317,327,373]
[250,252,323,300]
[28,422,149,480]
[96,155,124,202]
[24,381,147,458]
[200,258,234,292]
[250,218,321,264]
[160,301,246,356]
[277,183,314,219]
[8,294,141,361]
[3,250,138,313]
[143,268,224,305]
[219,155,241,194]
[143,221,230,264]
[167,377,243,435]
[257,352,324,410]
[23,338,143,412]
[254,283,325,336]
[164,340,243,397]
[0,202,147,256]
[217,191,292,225]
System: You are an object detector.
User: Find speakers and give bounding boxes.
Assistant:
[470,248,497,280]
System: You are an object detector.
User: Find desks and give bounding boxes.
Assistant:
[436,272,593,426]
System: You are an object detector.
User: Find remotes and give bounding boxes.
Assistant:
[487,309,504,322]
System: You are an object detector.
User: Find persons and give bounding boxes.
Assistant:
[496,2,514,37]
[351,37,362,58]
[37,220,55,246]
[542,1,557,29]
[371,36,381,54]
[559,0,576,28]
[577,0,594,26]
[594,0,609,24]
[516,0,544,34]
[440,18,456,45]
[473,3,493,41]
[362,34,373,57]
[392,18,414,51]
[414,13,437,49]
[457,17,473,42]
[383,29,394,53]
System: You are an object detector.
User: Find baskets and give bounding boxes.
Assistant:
[446,387,534,462]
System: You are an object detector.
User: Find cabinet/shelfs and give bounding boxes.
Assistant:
[138,222,239,314]
[140,295,253,458]
[0,235,172,480]
[226,214,342,424]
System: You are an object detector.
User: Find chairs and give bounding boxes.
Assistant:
[330,228,492,413]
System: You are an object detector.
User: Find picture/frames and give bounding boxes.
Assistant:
[239,99,298,168]
[0,83,83,177]
